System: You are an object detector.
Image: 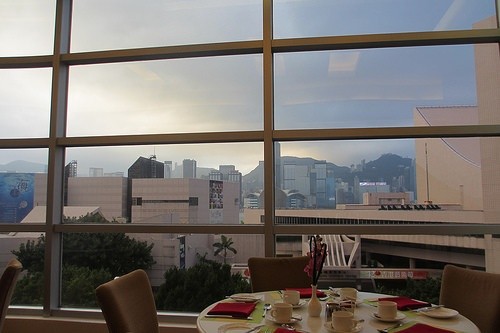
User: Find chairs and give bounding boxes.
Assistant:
[95,269,159,333]
[0,259,22,333]
[440,265,500,333]
[247,256,310,293]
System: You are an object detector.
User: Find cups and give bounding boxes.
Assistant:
[281,291,300,305]
[377,301,397,318]
[332,311,358,331]
[340,288,357,303]
[271,303,293,321]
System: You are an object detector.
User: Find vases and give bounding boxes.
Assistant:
[309,285,321,316]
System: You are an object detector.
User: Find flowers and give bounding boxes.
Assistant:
[304,235,327,285]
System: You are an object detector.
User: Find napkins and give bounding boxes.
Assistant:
[275,328,300,333]
[207,302,255,317]
[399,323,454,333]
[286,288,327,298]
[379,297,429,311]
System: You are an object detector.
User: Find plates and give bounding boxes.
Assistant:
[324,321,364,333]
[267,315,300,324]
[275,300,305,308]
[419,307,459,318]
[369,309,405,322]
[218,322,260,333]
[331,297,356,302]
[330,288,358,296]
[231,293,263,302]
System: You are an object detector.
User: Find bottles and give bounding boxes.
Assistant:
[326,300,356,320]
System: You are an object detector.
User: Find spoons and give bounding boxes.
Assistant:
[377,323,400,332]
[262,303,271,317]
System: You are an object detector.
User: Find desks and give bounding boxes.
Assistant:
[196,289,481,333]
